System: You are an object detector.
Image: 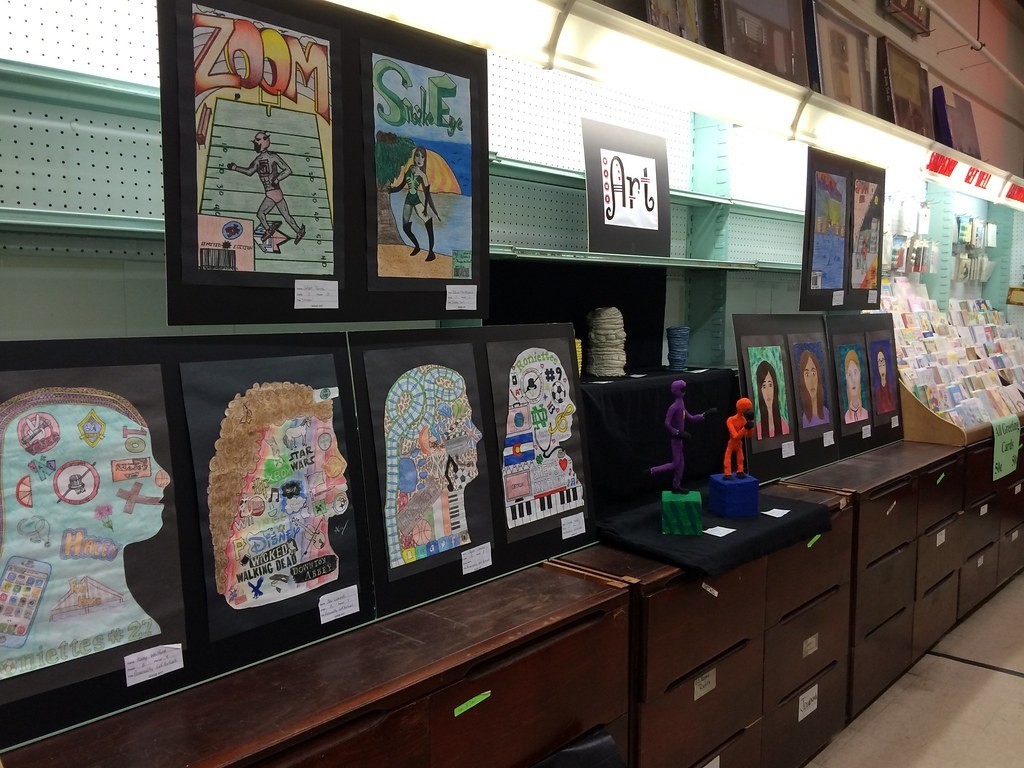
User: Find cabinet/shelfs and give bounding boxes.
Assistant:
[1,57,516,258]
[550,430,1024,768]
[729,198,890,273]
[490,157,760,270]
[1,562,630,768]
